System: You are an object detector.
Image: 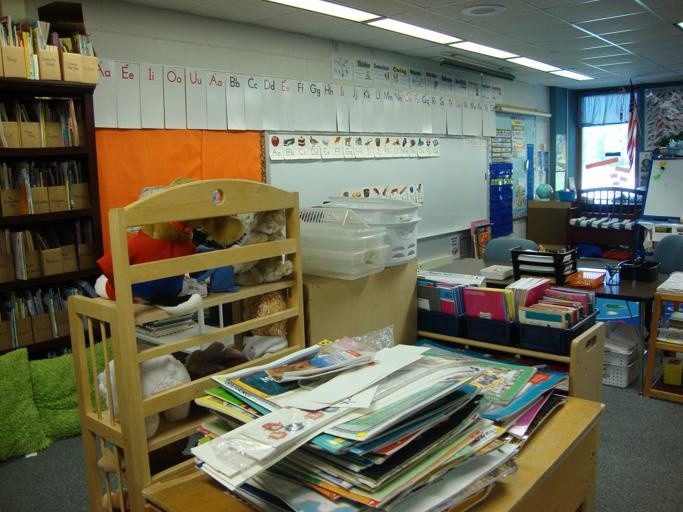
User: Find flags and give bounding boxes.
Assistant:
[626,82,639,169]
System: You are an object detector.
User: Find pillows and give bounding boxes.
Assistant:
[27,336,113,442]
[0,346,54,464]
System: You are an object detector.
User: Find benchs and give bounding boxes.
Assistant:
[565,187,645,260]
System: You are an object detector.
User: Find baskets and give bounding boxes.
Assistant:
[601,333,641,389]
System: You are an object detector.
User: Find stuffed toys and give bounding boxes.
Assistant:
[87,175,294,511]
[94,178,294,511]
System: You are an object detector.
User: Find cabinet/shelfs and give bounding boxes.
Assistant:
[0,1,103,361]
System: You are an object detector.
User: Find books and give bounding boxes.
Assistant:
[187,336,569,512]
[668,322,682,329]
[668,311,682,323]
[417,249,597,327]
[0,12,97,323]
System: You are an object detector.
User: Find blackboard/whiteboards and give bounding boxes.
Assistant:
[641,157,683,220]
[264,130,490,256]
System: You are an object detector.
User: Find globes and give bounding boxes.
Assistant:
[536,184,554,201]
